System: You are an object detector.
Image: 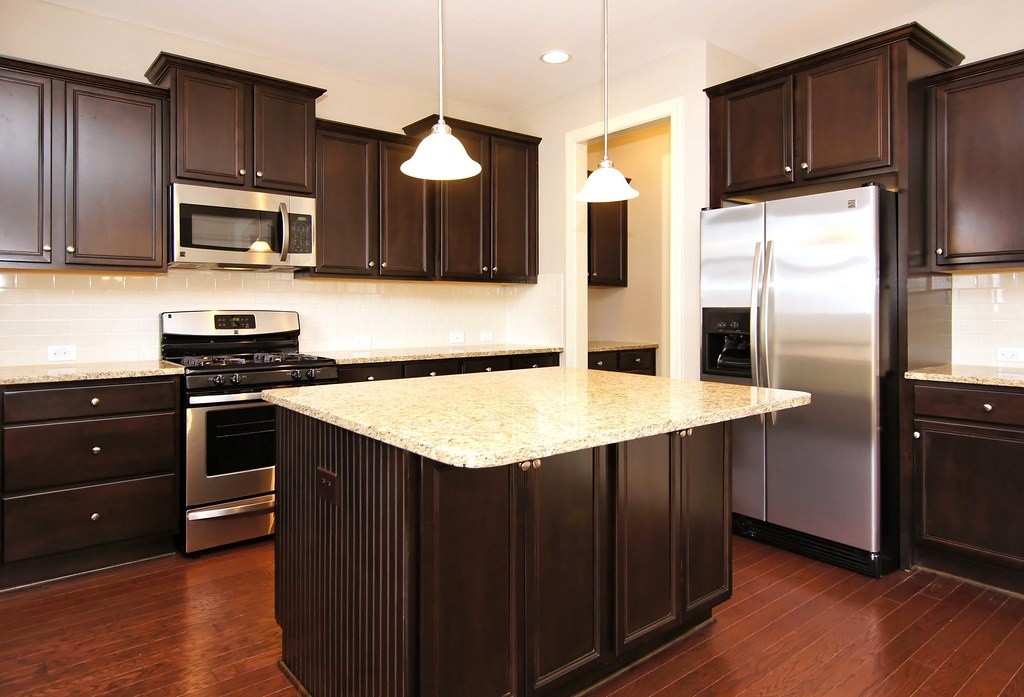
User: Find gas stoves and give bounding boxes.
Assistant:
[161,310,339,393]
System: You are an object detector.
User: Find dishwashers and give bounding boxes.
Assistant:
[185,392,276,553]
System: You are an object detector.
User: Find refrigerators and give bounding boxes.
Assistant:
[701,182,900,579]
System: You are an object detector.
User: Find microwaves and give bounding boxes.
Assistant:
[168,183,316,274]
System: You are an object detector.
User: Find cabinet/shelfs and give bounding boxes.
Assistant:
[1,50,542,285]
[902,381,1024,593]
[588,348,656,377]
[269,404,732,697]
[339,354,560,382]
[702,23,1023,275]
[588,170,632,286]
[0,376,185,592]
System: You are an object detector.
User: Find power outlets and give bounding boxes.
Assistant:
[48,344,80,362]
[448,332,464,343]
[480,331,494,340]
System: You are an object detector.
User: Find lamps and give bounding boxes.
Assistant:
[401,0,483,180]
[573,0,638,202]
